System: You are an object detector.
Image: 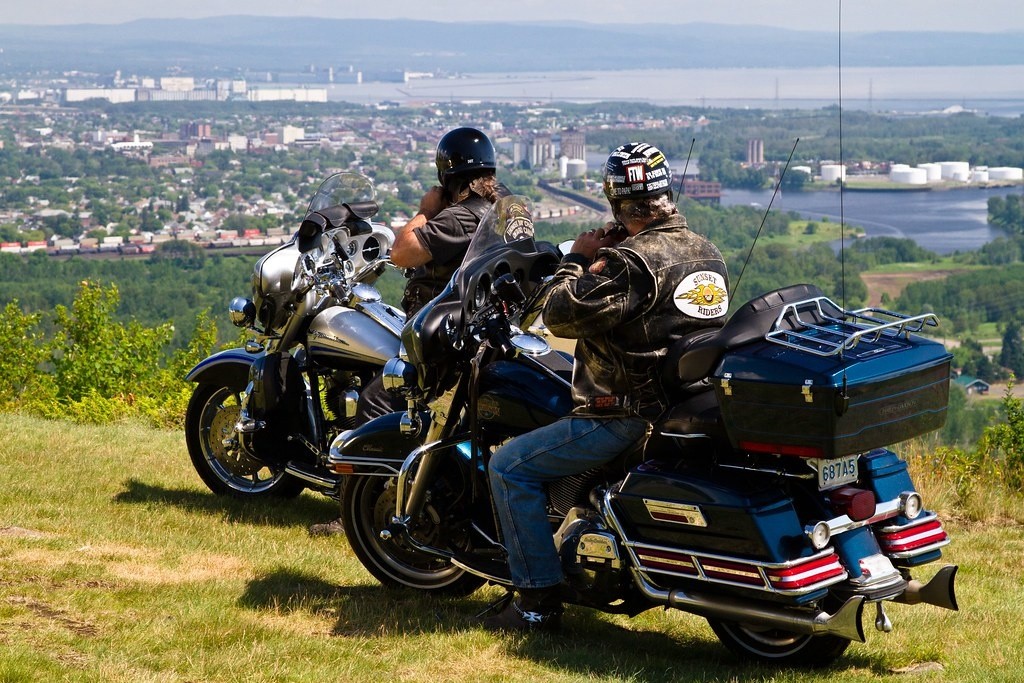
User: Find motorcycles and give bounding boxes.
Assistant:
[181,173,409,510]
[328,193,961,669]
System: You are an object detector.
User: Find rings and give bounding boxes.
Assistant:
[587,229,594,234]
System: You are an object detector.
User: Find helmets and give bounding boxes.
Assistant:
[433,124,497,176]
[602,140,674,201]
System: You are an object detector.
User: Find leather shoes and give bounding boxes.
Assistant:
[476,593,564,637]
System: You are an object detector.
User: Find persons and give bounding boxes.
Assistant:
[389,127,515,331]
[486,142,729,632]
